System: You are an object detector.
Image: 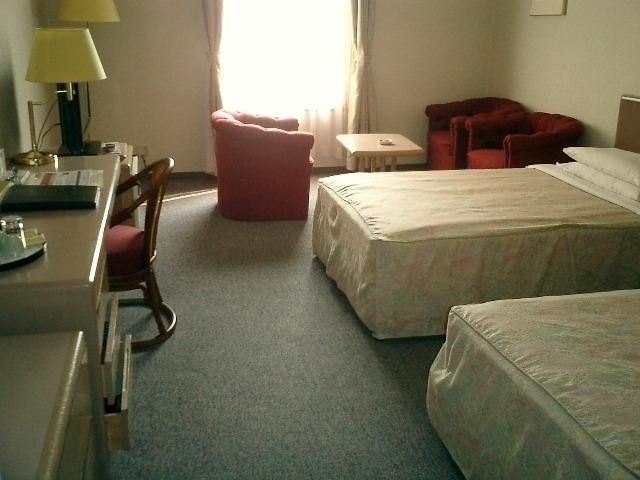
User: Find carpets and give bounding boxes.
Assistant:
[91,186,461,478]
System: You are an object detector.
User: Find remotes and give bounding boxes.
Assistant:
[106,144,115,151]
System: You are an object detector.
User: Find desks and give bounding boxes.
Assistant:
[0,152,138,452]
[1,328,114,480]
[335,131,426,171]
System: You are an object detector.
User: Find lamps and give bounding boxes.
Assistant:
[12,24,110,165]
[52,0,122,139]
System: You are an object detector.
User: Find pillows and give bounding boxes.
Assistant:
[562,146,639,189]
[555,160,639,205]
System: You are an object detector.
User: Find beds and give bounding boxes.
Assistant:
[424,285,638,478]
[310,92,638,343]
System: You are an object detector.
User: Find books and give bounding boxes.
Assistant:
[0,185,101,212]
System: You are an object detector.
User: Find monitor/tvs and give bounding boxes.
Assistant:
[57,82,101,156]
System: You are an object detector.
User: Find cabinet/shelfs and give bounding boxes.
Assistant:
[101,141,140,231]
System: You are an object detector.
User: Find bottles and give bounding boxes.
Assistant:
[0,215,26,256]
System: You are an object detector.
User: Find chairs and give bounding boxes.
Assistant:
[101,154,177,354]
[424,97,520,170]
[462,109,585,169]
[207,106,315,222]
